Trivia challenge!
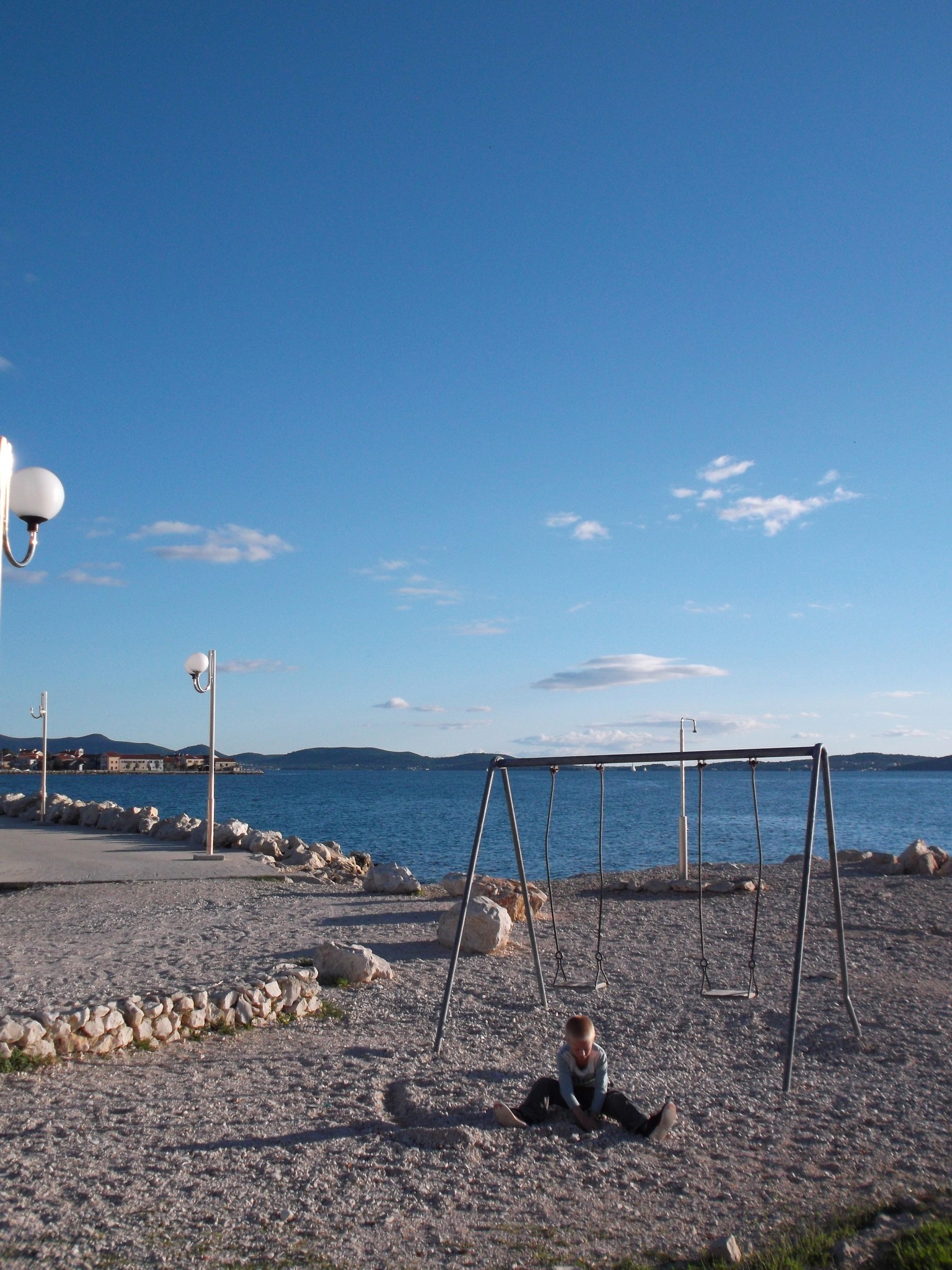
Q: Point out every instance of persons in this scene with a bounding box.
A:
[491,1015,677,1145]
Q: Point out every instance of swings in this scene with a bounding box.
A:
[543,767,610,995]
[698,758,762,1000]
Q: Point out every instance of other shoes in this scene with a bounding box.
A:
[640,1101,677,1143]
[494,1100,529,1129]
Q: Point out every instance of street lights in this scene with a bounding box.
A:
[678,717,698,880]
[184,649,216,855]
